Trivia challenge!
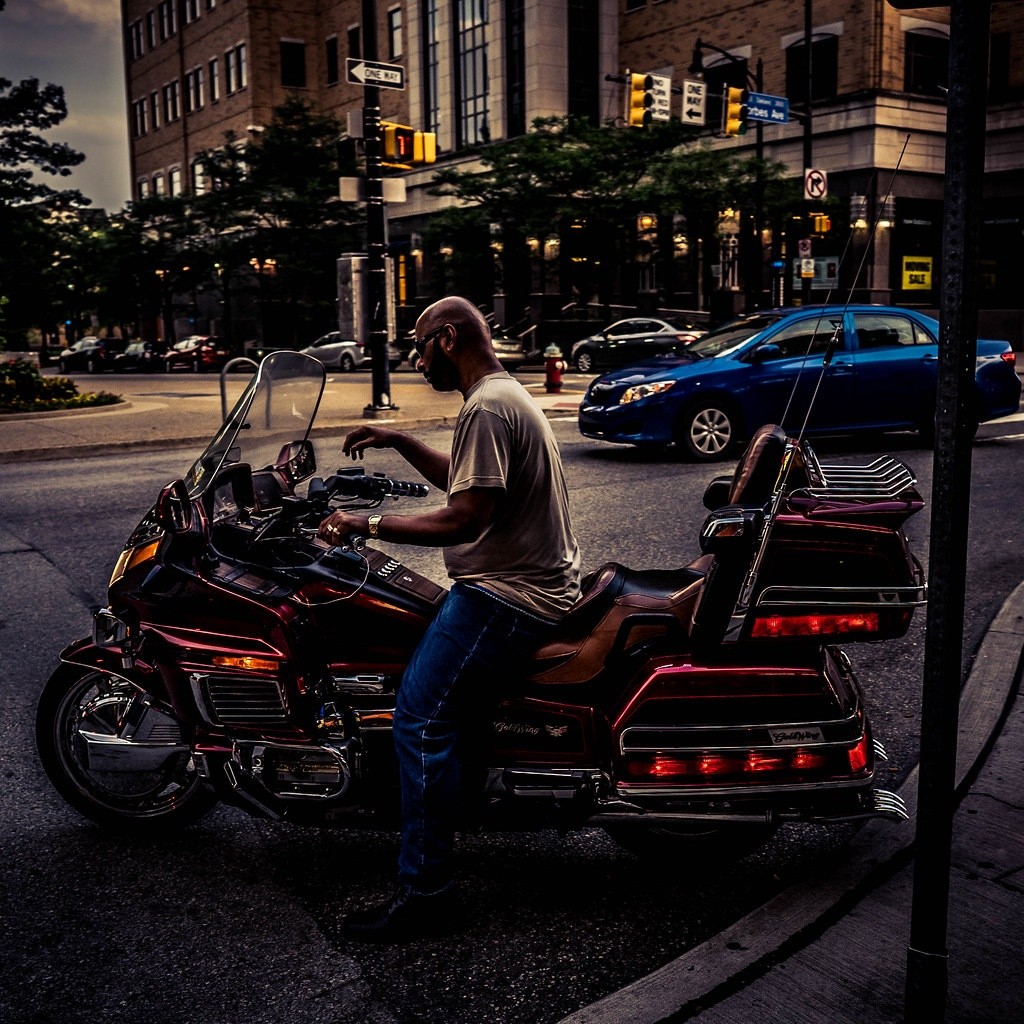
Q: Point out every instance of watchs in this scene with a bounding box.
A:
[367,514,383,539]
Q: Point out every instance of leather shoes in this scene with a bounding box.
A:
[340,883,466,944]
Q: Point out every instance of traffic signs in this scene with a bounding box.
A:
[345,57,407,93]
[683,78,707,125]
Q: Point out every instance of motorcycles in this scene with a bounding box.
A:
[31,346,918,863]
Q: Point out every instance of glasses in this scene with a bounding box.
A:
[412,324,462,357]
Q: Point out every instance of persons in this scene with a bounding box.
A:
[319,295,581,942]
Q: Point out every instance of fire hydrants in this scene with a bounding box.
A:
[541,342,571,393]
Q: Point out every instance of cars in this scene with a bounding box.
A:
[294,332,403,373]
[573,304,1024,458]
[568,315,708,375]
[37,346,67,368]
[162,335,234,374]
[113,340,169,374]
[405,332,527,370]
[58,335,124,374]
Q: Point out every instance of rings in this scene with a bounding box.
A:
[333,527,341,534]
[327,524,334,531]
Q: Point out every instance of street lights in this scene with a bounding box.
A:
[688,36,765,312]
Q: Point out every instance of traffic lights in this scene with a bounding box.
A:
[721,82,751,139]
[815,214,832,233]
[384,126,416,161]
[623,69,658,130]
[413,131,437,163]
